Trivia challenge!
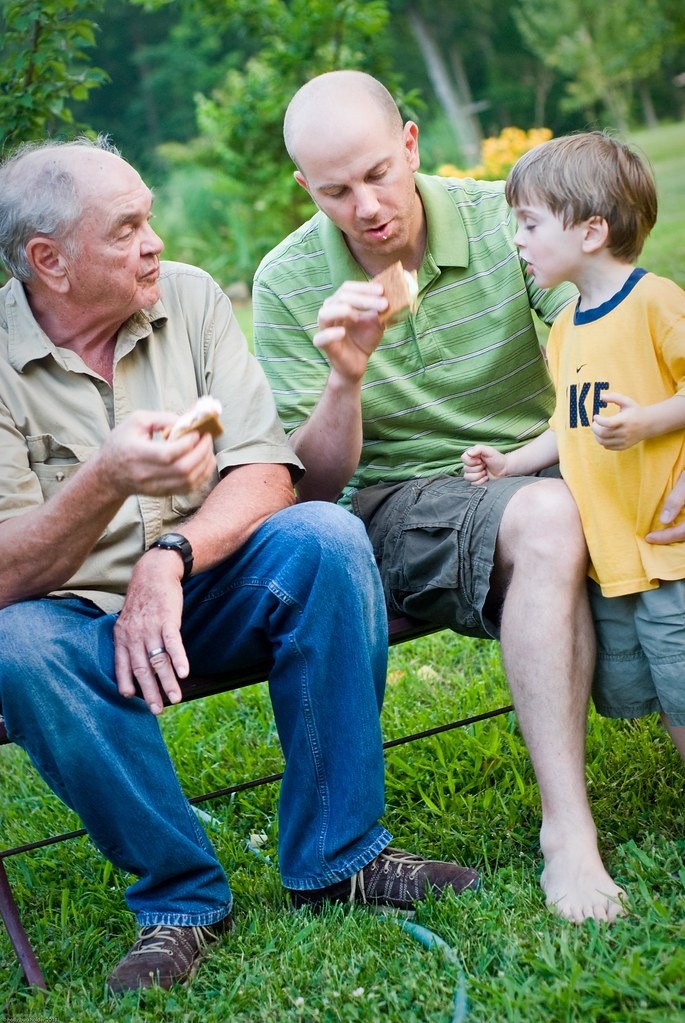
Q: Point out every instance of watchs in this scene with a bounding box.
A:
[148,533,193,583]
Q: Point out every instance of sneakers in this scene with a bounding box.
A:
[108,903,234,998]
[289,845,478,912]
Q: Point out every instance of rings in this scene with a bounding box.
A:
[148,648,167,658]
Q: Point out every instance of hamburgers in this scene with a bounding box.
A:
[372,262,419,324]
[162,393,223,441]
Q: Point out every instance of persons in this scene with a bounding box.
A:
[249,70,685,925]
[0,144,485,990]
[461,126,685,760]
